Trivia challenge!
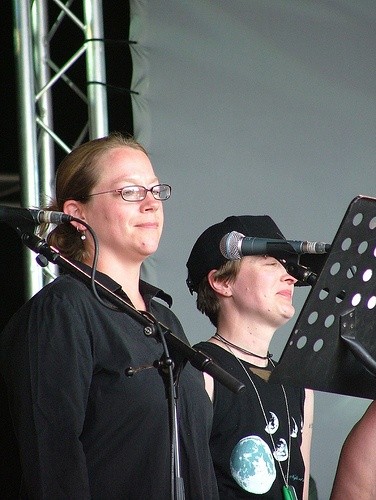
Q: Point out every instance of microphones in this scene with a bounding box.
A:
[220,230,331,261]
[0,205,73,225]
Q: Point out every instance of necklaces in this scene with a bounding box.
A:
[211,329,298,500]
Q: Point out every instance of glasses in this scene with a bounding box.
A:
[79,183,172,203]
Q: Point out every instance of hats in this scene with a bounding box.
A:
[186,215,330,295]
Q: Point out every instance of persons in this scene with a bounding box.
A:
[0,132,222,500]
[328,398,376,500]
[186,214,315,500]
